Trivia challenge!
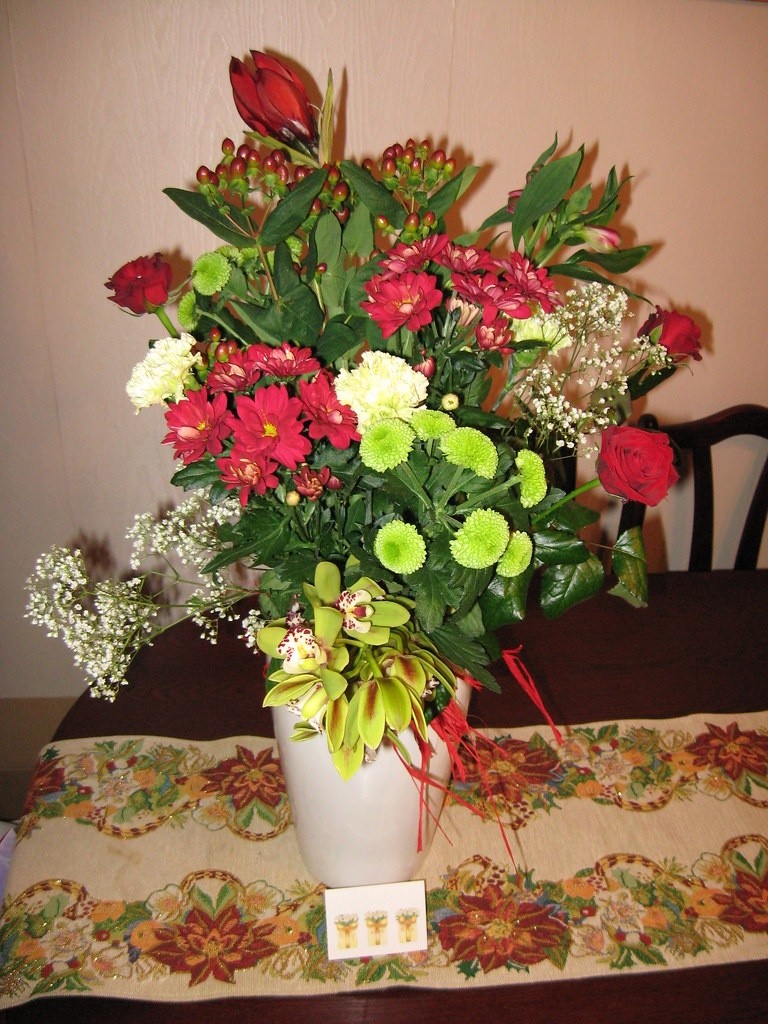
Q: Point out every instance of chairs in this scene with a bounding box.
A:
[616,403,768,569]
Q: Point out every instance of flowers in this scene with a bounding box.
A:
[22,51,707,786]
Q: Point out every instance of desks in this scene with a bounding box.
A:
[0,569,768,1024]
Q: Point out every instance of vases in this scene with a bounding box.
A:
[272,678,469,884]
